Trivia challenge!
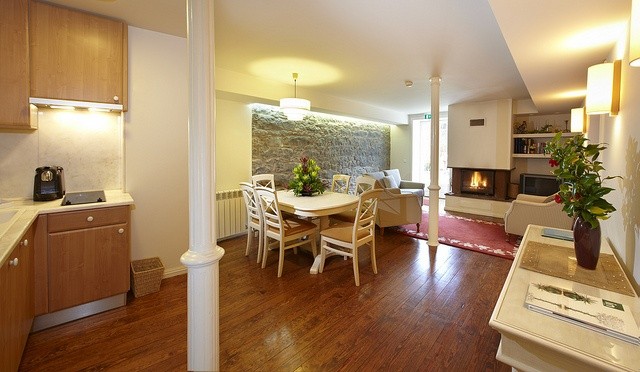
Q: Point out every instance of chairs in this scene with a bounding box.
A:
[310,174,352,251]
[256,189,318,278]
[363,173,421,235]
[316,189,384,286]
[252,174,276,248]
[328,174,375,260]
[238,181,297,263]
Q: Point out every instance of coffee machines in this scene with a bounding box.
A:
[33,165,66,202]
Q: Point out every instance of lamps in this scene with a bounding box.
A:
[585,60,621,117]
[279,73,310,120]
[570,106,586,134]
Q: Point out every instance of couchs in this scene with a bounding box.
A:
[503,188,574,242]
[365,169,424,206]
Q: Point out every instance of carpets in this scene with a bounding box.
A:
[371,212,522,260]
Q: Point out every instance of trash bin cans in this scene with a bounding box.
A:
[130,256,165,298]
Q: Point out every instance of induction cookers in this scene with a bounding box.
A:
[61,190,106,206]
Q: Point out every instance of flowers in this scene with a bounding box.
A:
[543,131,623,229]
[287,155,325,196]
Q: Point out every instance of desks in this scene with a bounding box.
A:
[276,187,360,256]
[487,224,640,372]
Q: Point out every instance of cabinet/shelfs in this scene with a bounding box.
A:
[0,0,39,130]
[27,0,128,113]
[34,190,134,331]
[0,213,39,372]
[513,113,581,198]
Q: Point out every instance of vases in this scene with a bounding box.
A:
[573,213,601,270]
[300,186,313,196]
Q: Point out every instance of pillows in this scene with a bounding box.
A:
[382,175,398,190]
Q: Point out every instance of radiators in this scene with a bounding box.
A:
[216,188,249,243]
